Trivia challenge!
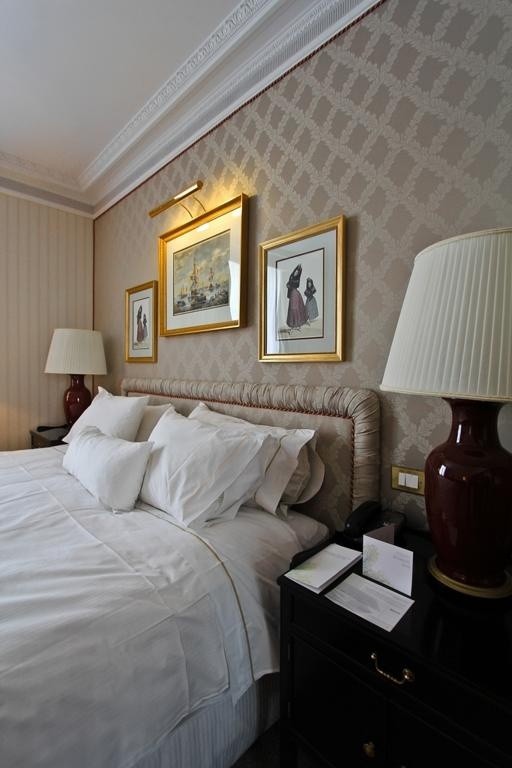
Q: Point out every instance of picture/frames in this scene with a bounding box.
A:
[259,214,346,364]
[124,277,157,364]
[158,192,251,339]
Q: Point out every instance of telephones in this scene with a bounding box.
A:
[344,501,408,548]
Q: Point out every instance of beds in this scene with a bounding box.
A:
[2,376,381,764]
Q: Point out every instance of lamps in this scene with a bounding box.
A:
[373,226,512,600]
[148,180,210,224]
[45,325,110,427]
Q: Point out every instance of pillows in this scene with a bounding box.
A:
[186,401,323,516]
[62,425,155,513]
[60,387,172,442]
[139,406,278,531]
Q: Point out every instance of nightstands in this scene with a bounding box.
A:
[28,422,71,447]
[279,513,511,765]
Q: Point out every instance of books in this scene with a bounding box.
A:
[284,522,415,633]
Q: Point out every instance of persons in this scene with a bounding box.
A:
[286,264,318,332]
[137,306,148,343]
